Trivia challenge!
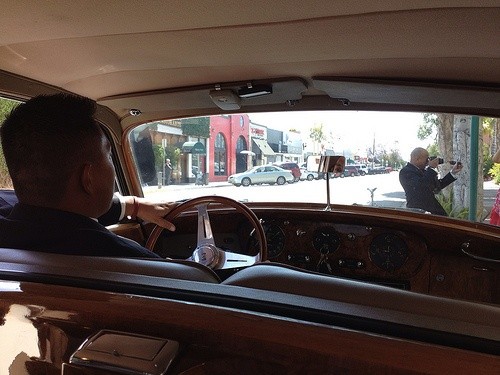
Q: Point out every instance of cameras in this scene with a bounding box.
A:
[429,155,444,167]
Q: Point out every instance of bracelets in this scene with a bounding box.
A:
[127,195,138,220]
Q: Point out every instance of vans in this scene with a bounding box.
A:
[266,162,301,182]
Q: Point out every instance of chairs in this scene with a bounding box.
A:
[0,248,500,328]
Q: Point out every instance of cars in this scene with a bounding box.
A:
[229,165,294,187]
[300,166,319,181]
[318,164,393,181]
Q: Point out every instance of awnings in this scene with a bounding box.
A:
[183,141,206,156]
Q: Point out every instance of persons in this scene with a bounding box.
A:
[489,148,500,226]
[332,156,345,173]
[0,92,182,260]
[164,159,172,186]
[399,147,463,216]
